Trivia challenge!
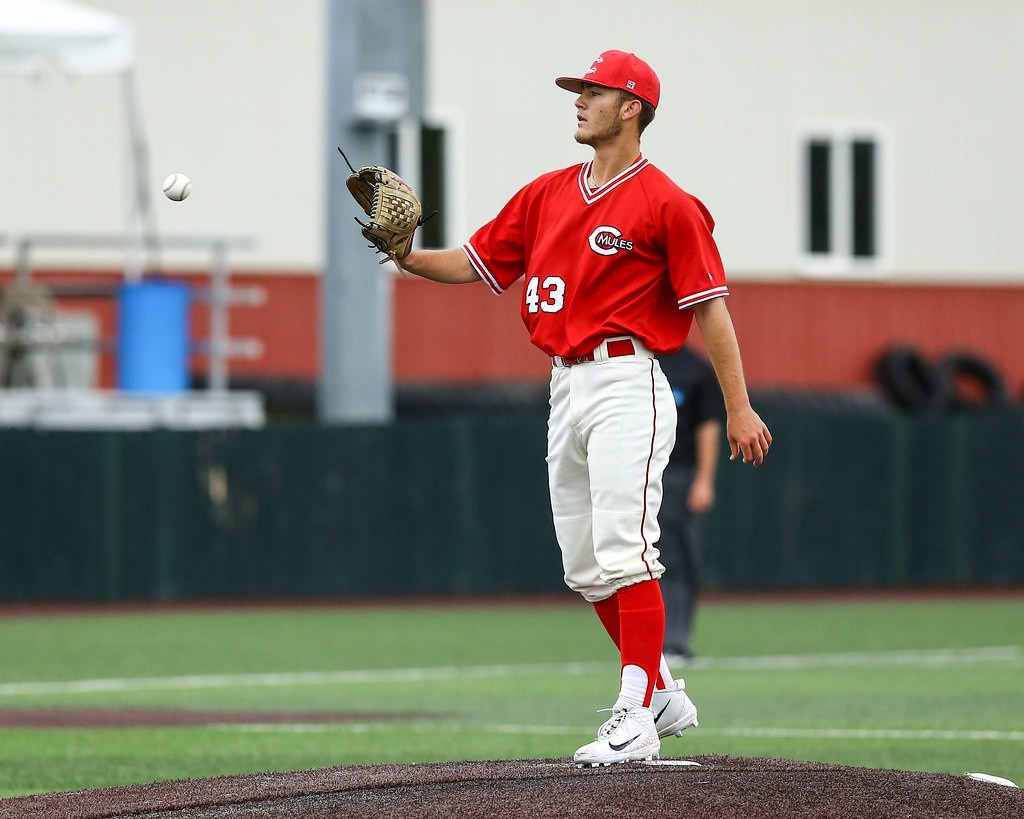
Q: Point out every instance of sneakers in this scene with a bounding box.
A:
[648,678,700,739]
[573,705,661,768]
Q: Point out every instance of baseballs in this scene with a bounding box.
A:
[163,173,192,202]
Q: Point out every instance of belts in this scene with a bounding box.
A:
[550,338,635,367]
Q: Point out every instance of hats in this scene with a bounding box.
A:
[555,49,660,110]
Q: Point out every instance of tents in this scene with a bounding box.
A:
[0,0,160,273]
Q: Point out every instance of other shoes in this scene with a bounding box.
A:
[664,651,691,669]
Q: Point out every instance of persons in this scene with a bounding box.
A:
[364,50,773,764]
[653,339,725,667]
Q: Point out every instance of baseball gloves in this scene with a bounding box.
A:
[346,166,421,260]
